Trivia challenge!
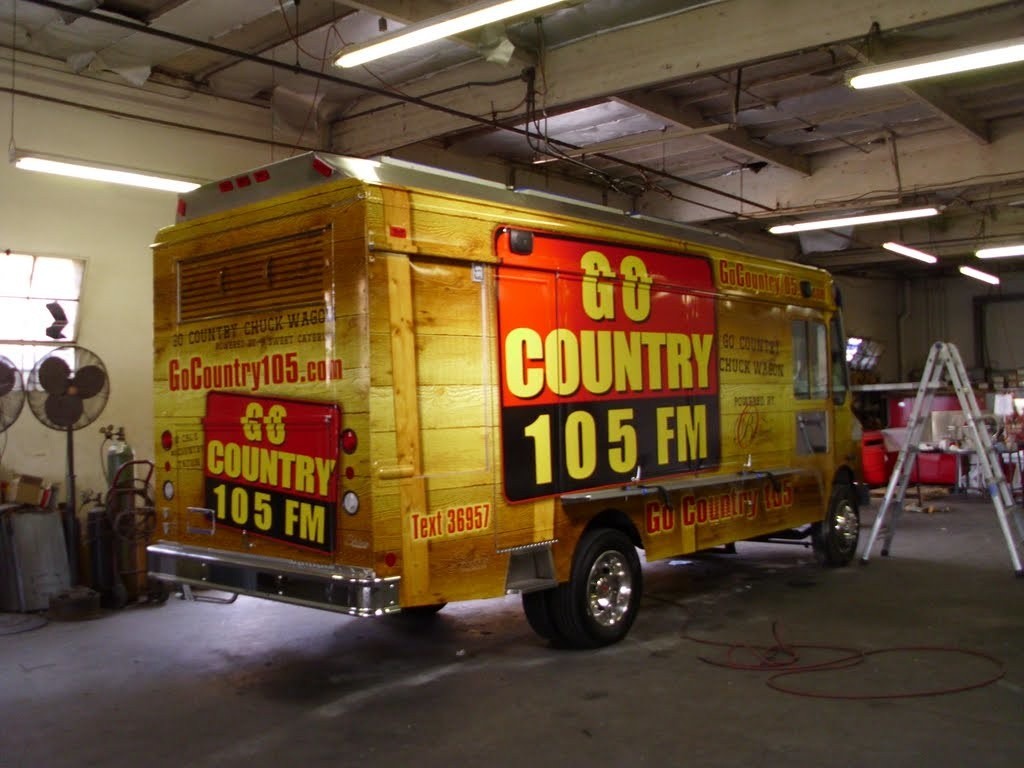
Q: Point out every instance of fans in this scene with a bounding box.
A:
[0,354,46,635]
[26,345,111,586]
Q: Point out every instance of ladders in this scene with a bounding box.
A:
[859,339,1024,580]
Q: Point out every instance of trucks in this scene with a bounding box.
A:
[143,146,883,651]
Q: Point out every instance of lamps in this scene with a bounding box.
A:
[532,66,743,165]
[843,19,1024,90]
[957,255,1000,285]
[4,1,276,193]
[331,0,566,70]
[975,241,1023,259]
[763,202,943,235]
[878,196,941,265]
